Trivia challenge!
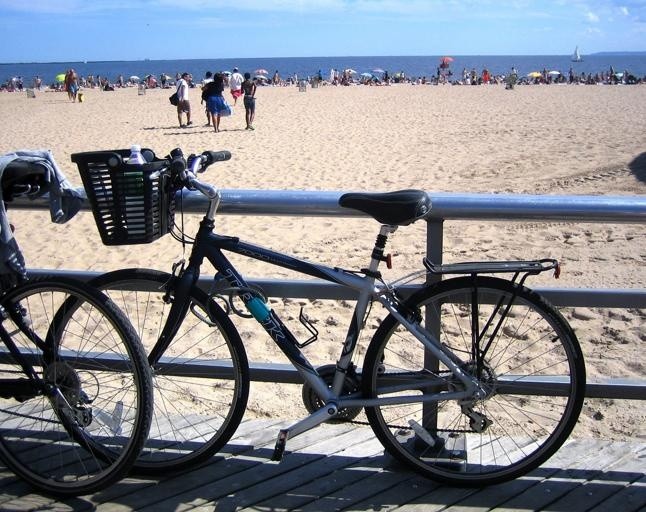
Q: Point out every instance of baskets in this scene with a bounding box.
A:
[71,148,176,245]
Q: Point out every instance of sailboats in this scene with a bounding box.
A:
[570,44,585,63]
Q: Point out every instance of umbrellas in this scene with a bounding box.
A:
[440,57,455,64]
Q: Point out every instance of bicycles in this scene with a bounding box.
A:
[0,159,157,502]
[36,145,590,488]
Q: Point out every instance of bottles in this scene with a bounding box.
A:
[124,146,146,233]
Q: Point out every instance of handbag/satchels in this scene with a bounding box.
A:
[169,92,179,105]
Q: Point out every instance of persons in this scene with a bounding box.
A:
[201,71,214,127]
[240,72,258,131]
[176,73,194,128]
[1,67,645,102]
[201,72,234,134]
